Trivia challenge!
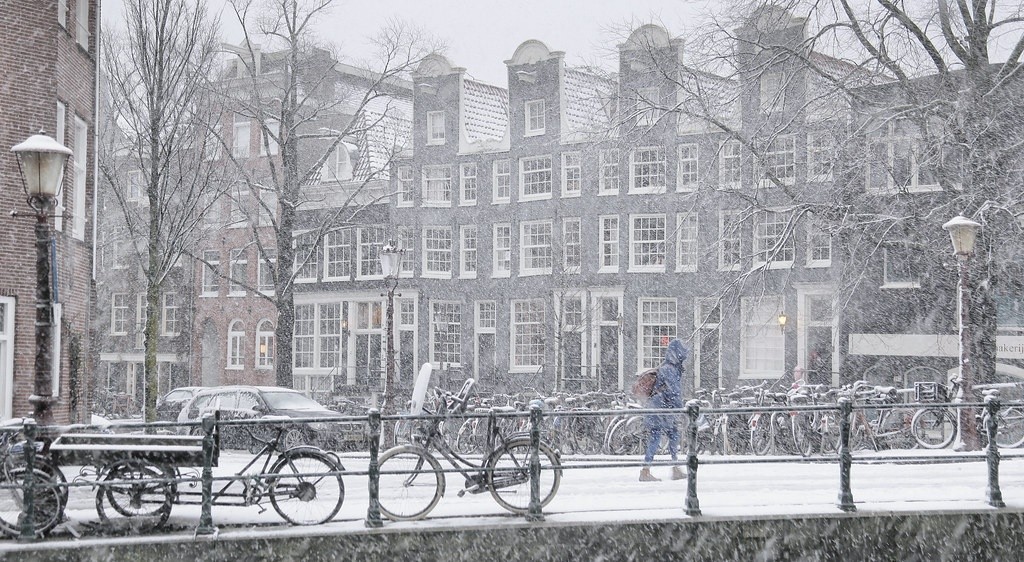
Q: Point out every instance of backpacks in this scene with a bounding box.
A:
[638,368,659,395]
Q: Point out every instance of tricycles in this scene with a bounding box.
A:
[43,407,348,536]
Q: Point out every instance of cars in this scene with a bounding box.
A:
[177,386,363,452]
[157,388,212,424]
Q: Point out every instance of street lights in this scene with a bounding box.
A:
[376,238,406,452]
[9,126,76,456]
[941,212,986,451]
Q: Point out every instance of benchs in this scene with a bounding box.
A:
[50,411,222,485]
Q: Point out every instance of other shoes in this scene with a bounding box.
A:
[673,467,688,479]
[639,470,662,481]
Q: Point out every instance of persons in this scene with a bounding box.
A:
[638,339,688,481]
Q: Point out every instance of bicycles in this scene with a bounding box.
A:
[326,378,924,458]
[910,374,1024,449]
[95,386,146,419]
[0,417,70,541]
[379,380,564,522]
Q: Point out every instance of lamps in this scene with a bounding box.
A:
[341,319,350,337]
[779,312,794,338]
[258,342,266,358]
